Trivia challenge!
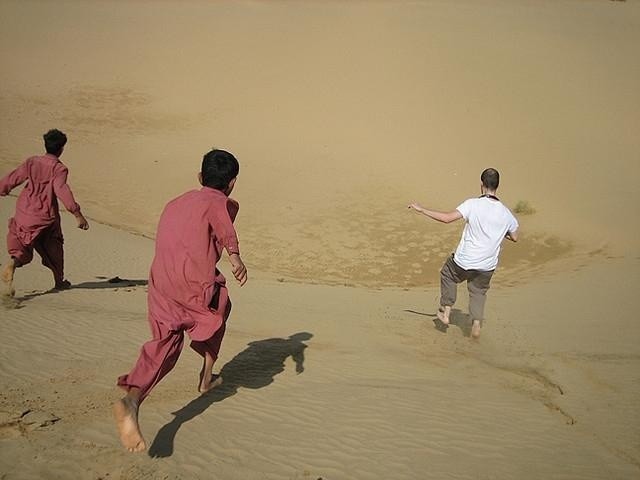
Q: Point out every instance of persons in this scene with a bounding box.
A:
[111,147,248,453]
[0,126,90,291]
[407,168,519,339]
